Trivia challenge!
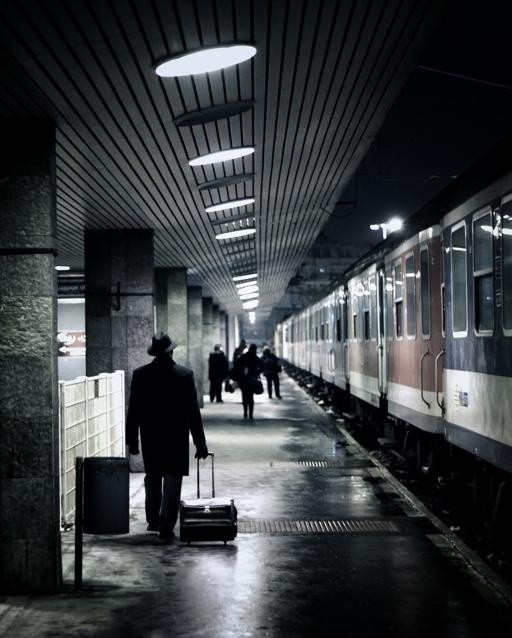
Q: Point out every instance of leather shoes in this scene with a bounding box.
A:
[147,523,175,541]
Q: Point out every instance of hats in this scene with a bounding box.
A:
[147,334,177,356]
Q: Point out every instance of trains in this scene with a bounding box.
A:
[273,146,510,529]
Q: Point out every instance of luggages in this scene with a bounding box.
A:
[179,452,238,544]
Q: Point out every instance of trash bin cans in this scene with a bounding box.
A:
[74,457,129,538]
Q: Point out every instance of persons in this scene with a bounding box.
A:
[259,348,282,399]
[232,339,246,366]
[237,343,265,419]
[125,332,208,542]
[209,343,230,404]
